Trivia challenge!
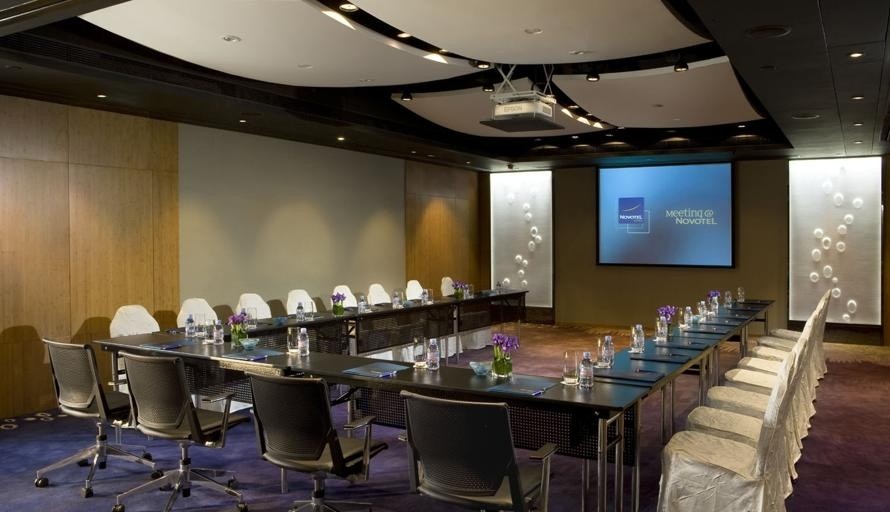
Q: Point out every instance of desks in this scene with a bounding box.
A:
[94,283,776,442]
[152,289,529,368]
[65,333,651,511]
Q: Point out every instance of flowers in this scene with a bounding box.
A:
[226,313,249,328]
[655,304,679,325]
[705,288,721,304]
[331,292,347,303]
[491,332,520,353]
[452,279,469,290]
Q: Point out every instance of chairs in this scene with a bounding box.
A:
[235,292,272,321]
[34,338,164,499]
[244,371,389,512]
[656,351,796,512]
[111,349,249,512]
[440,276,494,350]
[683,338,807,480]
[286,288,317,316]
[366,282,424,363]
[330,285,394,361]
[405,279,464,358]
[399,389,561,512]
[176,297,219,328]
[751,345,791,362]
[704,310,816,440]
[736,288,832,380]
[756,336,798,352]
[769,327,802,342]
[109,304,160,391]
[724,294,826,402]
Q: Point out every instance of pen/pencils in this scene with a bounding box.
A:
[165,344,182,351]
[251,355,268,360]
[690,341,704,344]
[381,371,397,377]
[533,388,546,396]
[667,353,688,357]
[637,369,658,372]
[168,330,182,333]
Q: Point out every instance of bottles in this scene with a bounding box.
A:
[496,281,502,295]
[426,338,440,370]
[710,297,718,318]
[421,289,428,305]
[601,335,615,367]
[464,285,469,300]
[357,296,365,314]
[578,351,594,388]
[240,308,248,331]
[659,316,668,338]
[393,292,399,309]
[296,302,304,321]
[297,328,310,357]
[700,301,708,322]
[186,314,196,337]
[737,288,742,302]
[683,306,693,329]
[634,324,644,354]
[213,320,224,345]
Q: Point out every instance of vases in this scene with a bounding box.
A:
[230,323,248,346]
[332,301,345,315]
[491,355,513,378]
[453,289,465,300]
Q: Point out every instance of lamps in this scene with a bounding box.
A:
[674,62,688,72]
[476,62,488,70]
[477,60,490,69]
[483,79,494,92]
[402,92,414,101]
[587,72,600,82]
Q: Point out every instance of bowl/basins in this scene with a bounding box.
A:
[470,361,493,376]
[238,338,259,350]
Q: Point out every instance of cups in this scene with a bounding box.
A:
[657,323,668,344]
[204,319,216,343]
[413,337,426,366]
[562,351,578,384]
[247,308,257,329]
[597,338,610,366]
[630,327,640,351]
[287,327,301,352]
[194,313,204,336]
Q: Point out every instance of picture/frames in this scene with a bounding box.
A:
[488,170,556,325]
[787,155,885,346]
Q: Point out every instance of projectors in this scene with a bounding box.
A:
[490,91,556,120]
